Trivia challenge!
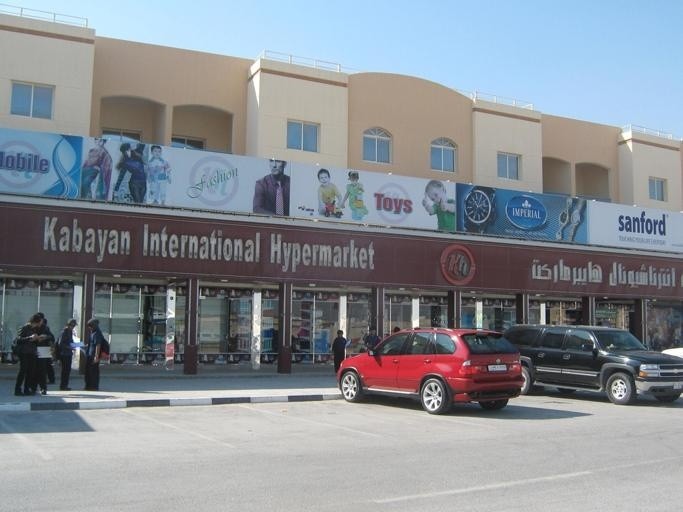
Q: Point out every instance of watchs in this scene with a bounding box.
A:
[463,186,498,233]
[555,197,573,241]
[567,199,585,242]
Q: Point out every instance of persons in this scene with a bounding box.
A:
[81,138,172,205]
[339,171,368,220]
[422,180,456,231]
[332,330,347,376]
[84,319,104,391]
[318,169,344,215]
[15,313,55,395]
[365,327,382,352]
[58,319,78,390]
[253,160,290,216]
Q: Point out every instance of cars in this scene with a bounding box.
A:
[661,347,683,358]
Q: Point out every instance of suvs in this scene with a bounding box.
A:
[503,319,682,407]
[335,326,526,414]
